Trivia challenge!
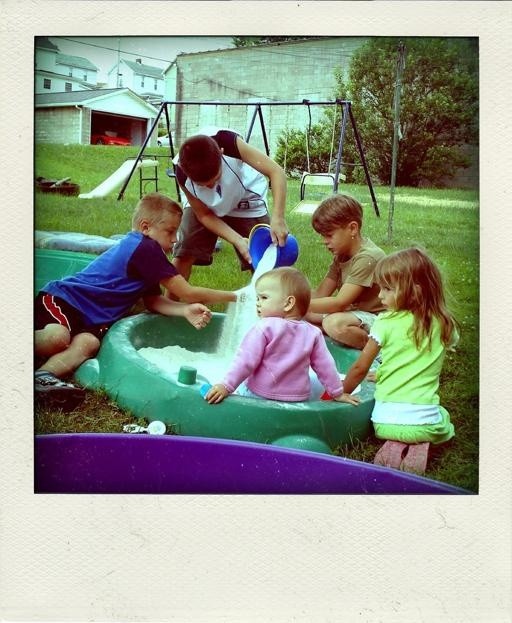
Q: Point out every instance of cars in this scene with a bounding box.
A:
[156,130,179,147]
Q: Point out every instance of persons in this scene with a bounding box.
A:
[341,247,462,476]
[33,194,247,408]
[206,267,361,406]
[162,129,290,305]
[303,193,388,350]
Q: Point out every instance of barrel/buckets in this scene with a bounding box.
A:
[247,223,298,275]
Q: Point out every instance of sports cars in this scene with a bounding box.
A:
[92,128,133,149]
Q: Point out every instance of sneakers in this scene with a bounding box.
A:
[373,440,407,470]
[34,370,85,412]
[399,443,429,476]
